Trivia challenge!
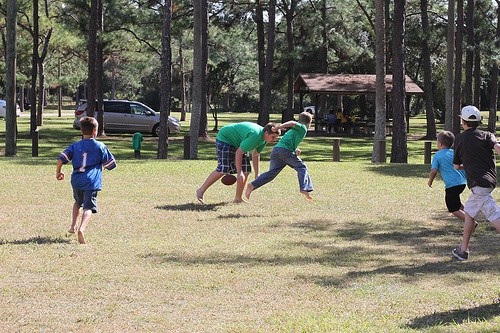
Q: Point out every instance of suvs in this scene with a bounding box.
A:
[72,100,182,137]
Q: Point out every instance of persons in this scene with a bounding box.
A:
[427,131,479,231]
[327,109,356,133]
[245,111,314,200]
[452,106,500,261]
[56,116,117,244]
[196,121,281,204]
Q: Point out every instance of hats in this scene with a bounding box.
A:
[458,106,481,121]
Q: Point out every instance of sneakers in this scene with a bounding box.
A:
[452,248,469,261]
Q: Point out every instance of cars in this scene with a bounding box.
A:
[0,99,22,119]
[303,106,320,113]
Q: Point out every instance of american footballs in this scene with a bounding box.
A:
[221,175,237,186]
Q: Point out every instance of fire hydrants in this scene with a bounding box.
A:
[132,132,143,160]
